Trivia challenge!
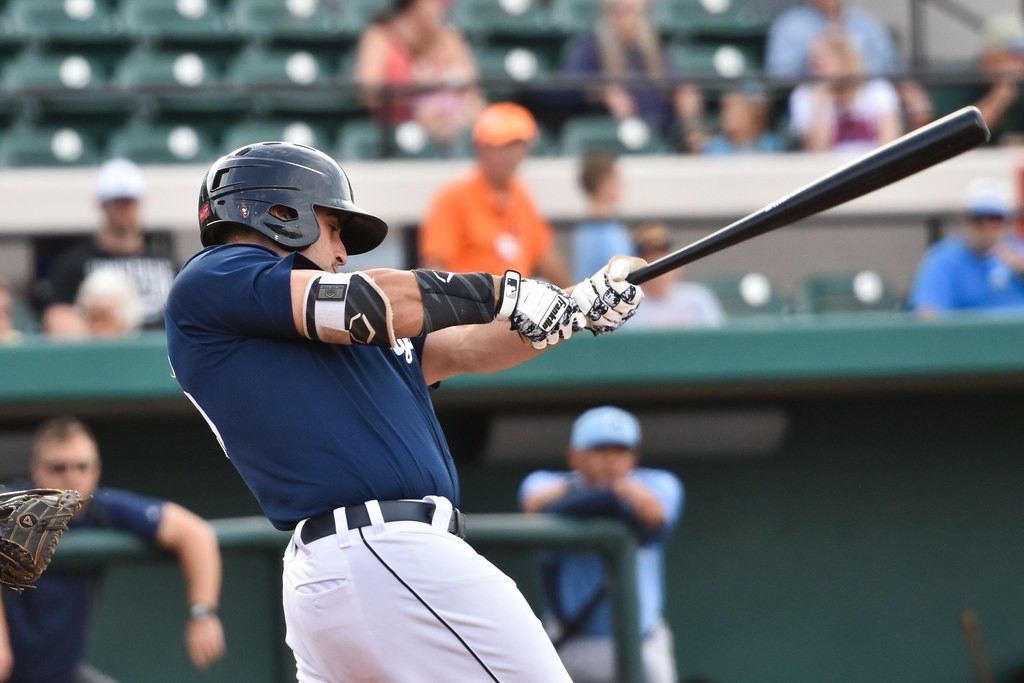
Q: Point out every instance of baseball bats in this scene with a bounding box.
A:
[627,106,991,285]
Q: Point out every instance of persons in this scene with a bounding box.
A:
[0,413,228,683]
[408,101,572,297]
[517,406,686,683]
[163,140,649,683]
[566,149,638,293]
[26,156,183,341]
[352,2,1023,159]
[608,219,729,329]
[902,182,1024,316]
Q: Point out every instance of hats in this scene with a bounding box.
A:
[476,102,537,146]
[98,158,143,202]
[571,406,641,448]
[966,181,1013,216]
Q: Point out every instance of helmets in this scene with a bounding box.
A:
[198,140,389,255]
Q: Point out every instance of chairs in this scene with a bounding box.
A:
[558,117,673,155]
[707,275,796,316]
[104,125,216,166]
[673,45,759,75]
[221,118,330,158]
[808,273,902,314]
[0,126,101,168]
[0,36,110,93]
[111,38,221,88]
[0,0,769,53]
[222,41,333,84]
[471,46,555,80]
[335,116,441,159]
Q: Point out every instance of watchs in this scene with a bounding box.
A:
[186,603,219,623]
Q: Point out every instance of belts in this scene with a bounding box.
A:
[294,501,468,556]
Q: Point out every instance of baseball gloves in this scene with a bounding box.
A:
[0,488,85,590]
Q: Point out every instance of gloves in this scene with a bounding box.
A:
[572,256,649,336]
[494,270,586,349]
